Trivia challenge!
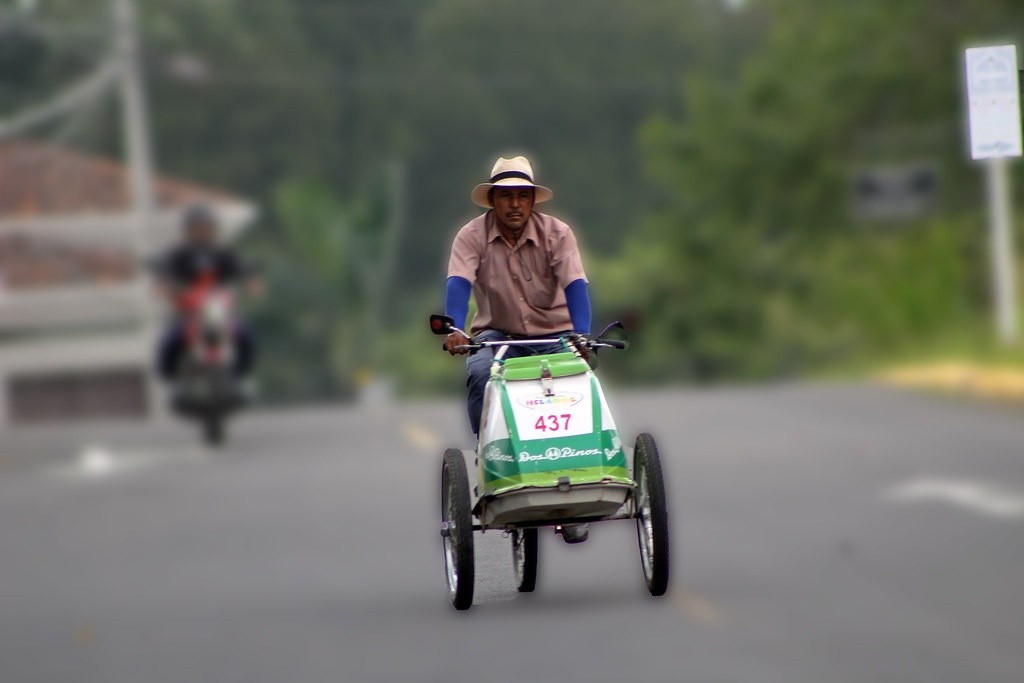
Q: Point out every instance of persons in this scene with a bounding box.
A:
[446,155,595,545]
[145,203,256,383]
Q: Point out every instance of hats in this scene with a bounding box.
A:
[472,156,553,209]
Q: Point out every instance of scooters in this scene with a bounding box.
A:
[157,251,257,444]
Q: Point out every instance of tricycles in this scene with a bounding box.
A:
[428,312,669,610]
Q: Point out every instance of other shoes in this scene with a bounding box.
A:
[562,523,588,542]
